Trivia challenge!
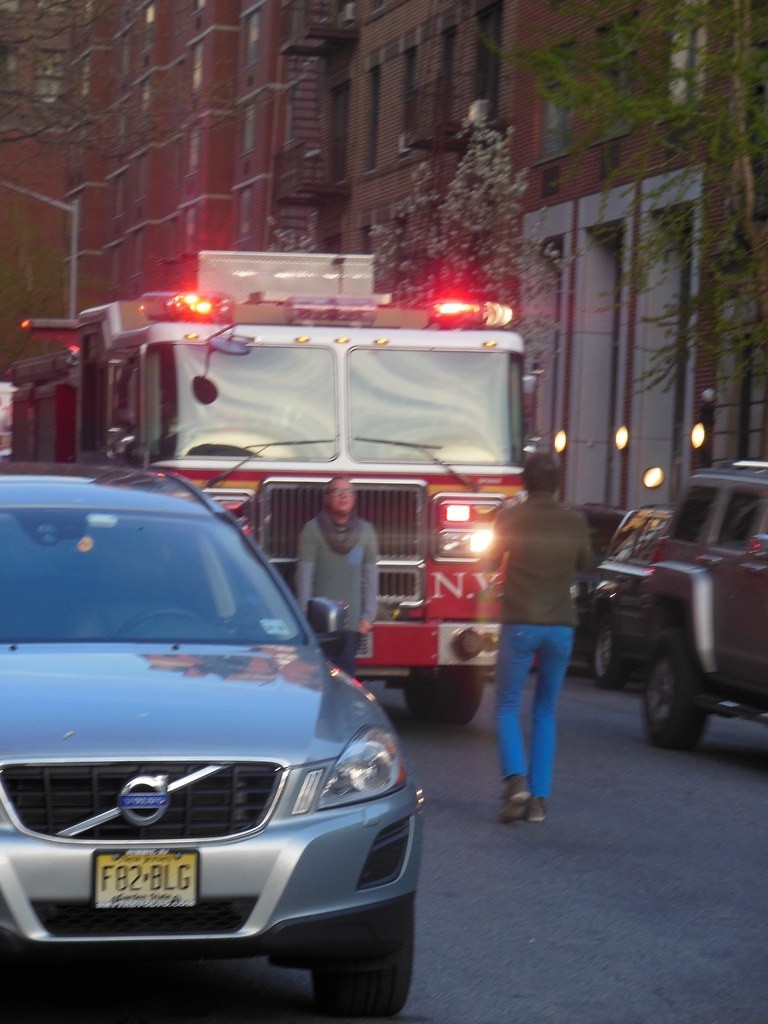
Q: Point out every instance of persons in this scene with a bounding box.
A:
[476,453,594,823]
[294,476,378,677]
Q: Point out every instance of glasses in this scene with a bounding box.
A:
[327,486,354,497]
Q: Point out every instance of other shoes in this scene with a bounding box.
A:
[499,776,531,822]
[526,797,549,822]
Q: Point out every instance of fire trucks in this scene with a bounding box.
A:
[7,249,527,729]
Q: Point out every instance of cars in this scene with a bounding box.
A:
[638,458,768,751]
[572,502,678,690]
[0,461,425,1017]
